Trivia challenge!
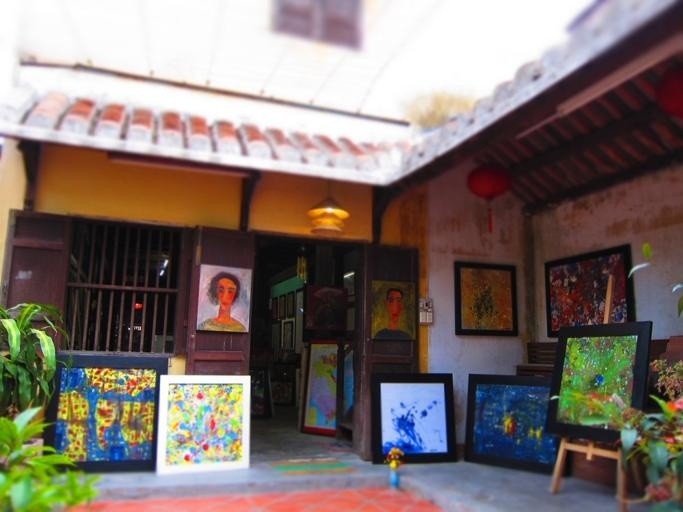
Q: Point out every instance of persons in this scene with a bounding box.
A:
[375,288,413,338]
[198,272,247,331]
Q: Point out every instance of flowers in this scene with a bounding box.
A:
[384,448,405,469]
[549,359,683,502]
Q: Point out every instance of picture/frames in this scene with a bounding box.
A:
[271,287,304,355]
[301,340,354,436]
[156,375,251,475]
[370,373,457,464]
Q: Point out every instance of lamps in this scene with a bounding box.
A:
[307,179,350,234]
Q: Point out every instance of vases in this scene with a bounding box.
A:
[390,470,399,487]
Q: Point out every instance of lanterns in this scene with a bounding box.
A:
[465,164,514,231]
